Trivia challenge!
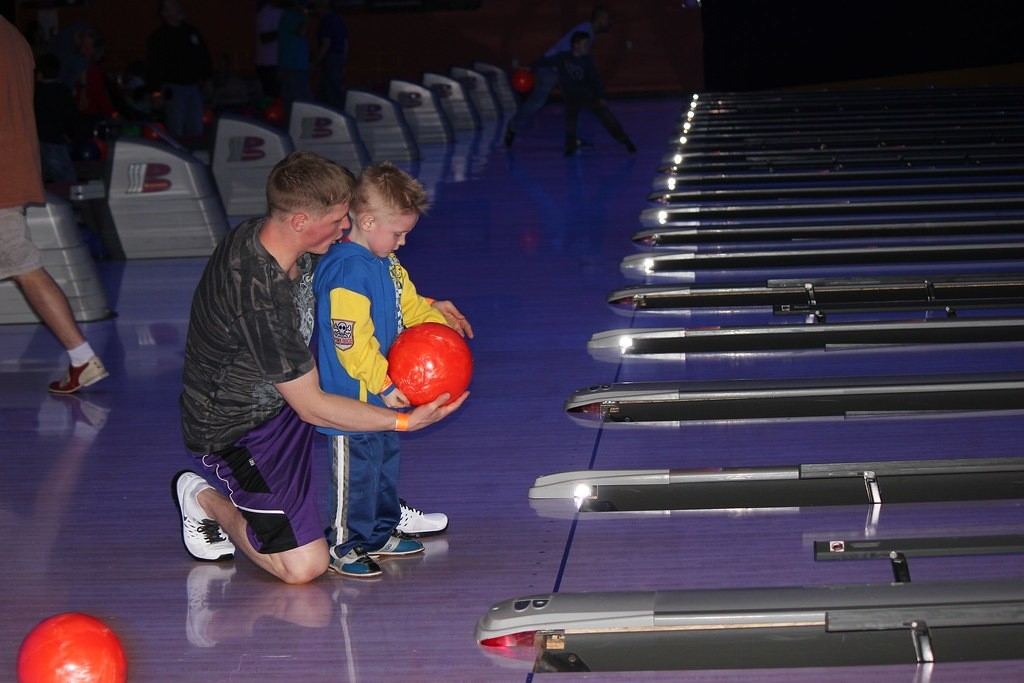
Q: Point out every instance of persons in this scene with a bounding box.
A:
[1,14,109,393]
[170,150,474,584]
[277,0,311,124]
[309,162,454,577]
[311,0,348,113]
[504,7,613,147]
[145,0,212,136]
[258,0,287,131]
[518,30,637,158]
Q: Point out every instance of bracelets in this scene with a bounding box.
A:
[394,411,408,432]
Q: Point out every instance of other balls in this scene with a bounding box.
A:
[90,138,108,161]
[267,105,286,122]
[201,108,214,125]
[16,613,129,683]
[512,69,535,92]
[387,322,473,407]
[144,121,167,141]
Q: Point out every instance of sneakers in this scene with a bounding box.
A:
[47,353,109,393]
[328,546,382,577]
[170,467,235,561]
[369,535,426,559]
[393,502,449,541]
